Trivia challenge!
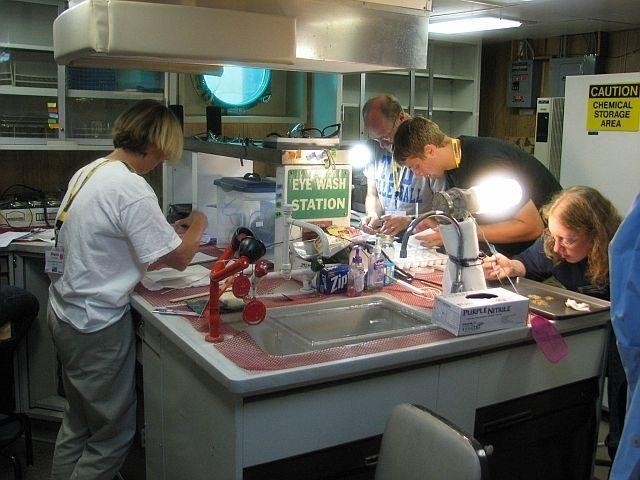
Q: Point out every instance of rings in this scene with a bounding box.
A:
[391,226,395,231]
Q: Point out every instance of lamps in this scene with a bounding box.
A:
[432,167,525,293]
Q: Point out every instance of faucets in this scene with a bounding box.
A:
[399,210,465,293]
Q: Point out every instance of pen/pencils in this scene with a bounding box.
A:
[489,244,497,269]
[152,307,204,317]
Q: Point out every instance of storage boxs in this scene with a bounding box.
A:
[213,176,274,259]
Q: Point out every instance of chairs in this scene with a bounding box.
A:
[0,285,41,479]
[375,402,495,480]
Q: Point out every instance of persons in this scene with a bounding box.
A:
[393,117,565,260]
[483,185,626,462]
[42,98,208,480]
[359,94,445,237]
[609,193,639,480]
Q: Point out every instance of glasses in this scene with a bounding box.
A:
[367,114,398,143]
[544,231,590,247]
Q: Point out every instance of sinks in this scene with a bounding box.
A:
[221,295,438,357]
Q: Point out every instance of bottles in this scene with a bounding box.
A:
[382,234,395,286]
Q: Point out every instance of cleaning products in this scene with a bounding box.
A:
[380,233,396,286]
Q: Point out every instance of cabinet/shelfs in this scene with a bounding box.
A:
[14,251,69,421]
[116,306,163,480]
[0,43,172,150]
[0,248,15,288]
[309,33,483,144]
